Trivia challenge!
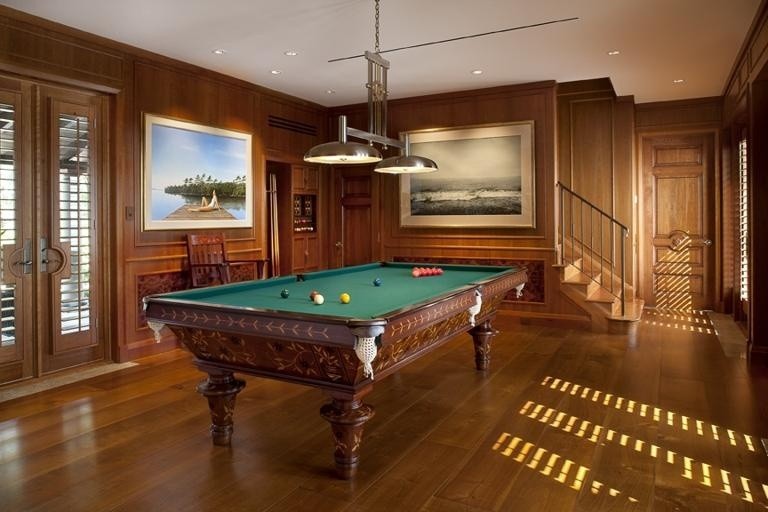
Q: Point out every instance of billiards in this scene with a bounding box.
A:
[412,267,443,277]
[310,291,324,305]
[340,293,351,303]
[281,289,289,297]
[374,278,383,286]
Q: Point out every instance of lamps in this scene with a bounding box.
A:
[303,0,439,175]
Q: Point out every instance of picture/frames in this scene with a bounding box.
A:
[139,111,254,232]
[397,119,536,230]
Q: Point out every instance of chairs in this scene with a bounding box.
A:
[188,232,268,289]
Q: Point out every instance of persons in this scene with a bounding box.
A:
[187,189,221,212]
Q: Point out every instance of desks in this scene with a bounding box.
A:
[142,260,529,479]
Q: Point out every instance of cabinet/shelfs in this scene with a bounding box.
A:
[280,165,322,274]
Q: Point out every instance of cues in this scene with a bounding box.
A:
[270,174,280,277]
